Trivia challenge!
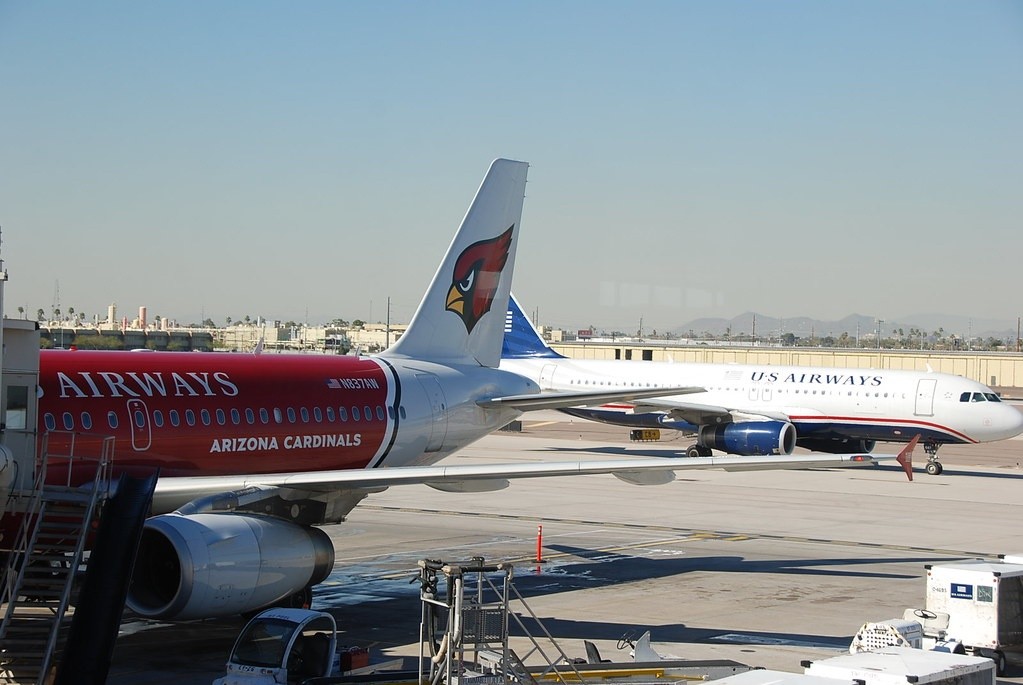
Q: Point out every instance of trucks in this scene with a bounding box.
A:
[851,558,1023,675]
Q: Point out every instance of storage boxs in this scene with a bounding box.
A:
[340,647,369,672]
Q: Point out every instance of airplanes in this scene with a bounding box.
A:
[501,291,1023,476]
[0,157,922,685]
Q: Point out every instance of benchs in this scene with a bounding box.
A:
[298,632,330,677]
